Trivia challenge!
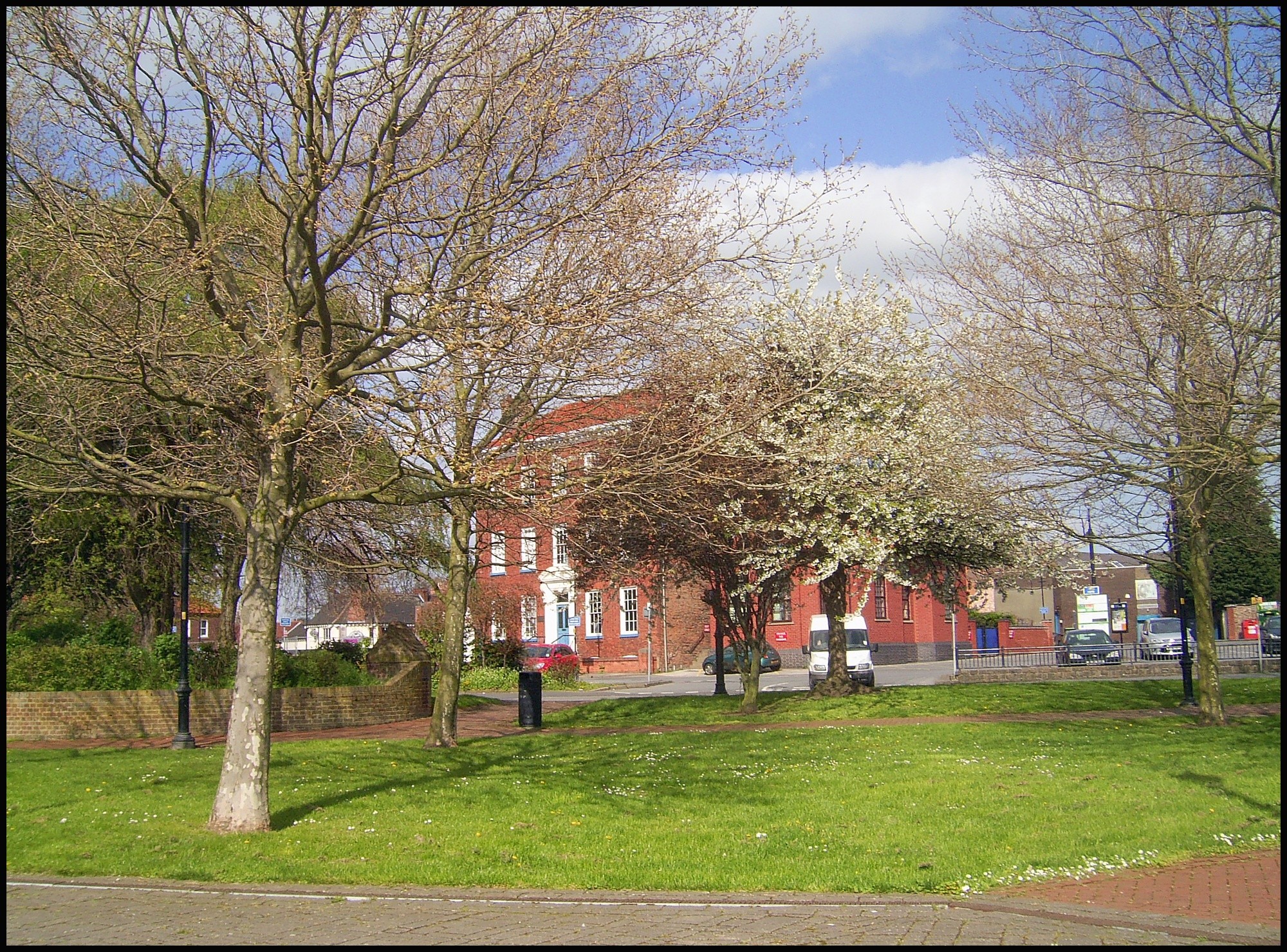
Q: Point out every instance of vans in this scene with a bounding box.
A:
[1139,617,1195,660]
[802,612,878,692]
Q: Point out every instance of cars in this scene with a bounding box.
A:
[1055,629,1121,666]
[702,639,781,675]
[1266,615,1280,653]
[515,643,579,673]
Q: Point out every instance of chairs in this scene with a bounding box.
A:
[1070,638,1078,642]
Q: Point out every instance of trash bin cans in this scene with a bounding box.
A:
[1241,619,1259,639]
[518,672,543,728]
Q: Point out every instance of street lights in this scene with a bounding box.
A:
[1156,427,1198,707]
[1083,494,1097,585]
